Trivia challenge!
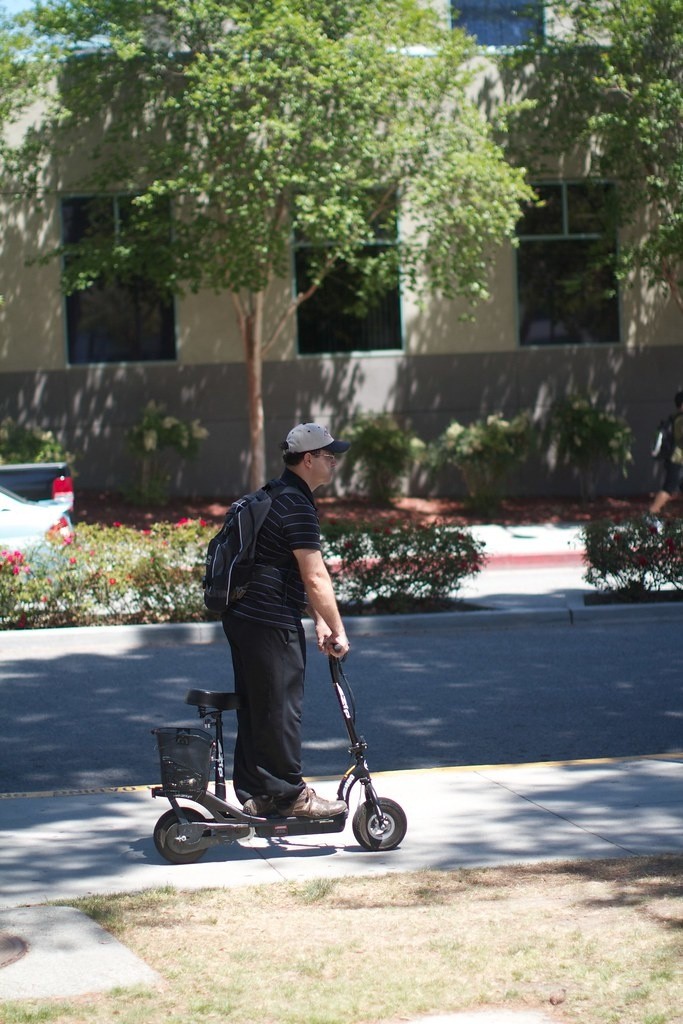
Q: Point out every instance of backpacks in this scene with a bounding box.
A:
[203,484,312,614]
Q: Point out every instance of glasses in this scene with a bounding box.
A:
[309,452,336,463]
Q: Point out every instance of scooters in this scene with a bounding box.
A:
[152,643,408,865]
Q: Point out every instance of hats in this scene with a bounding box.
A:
[284,423,351,458]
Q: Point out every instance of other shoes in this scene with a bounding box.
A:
[244,796,278,816]
[278,786,347,819]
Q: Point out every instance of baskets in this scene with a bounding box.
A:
[151,726,212,795]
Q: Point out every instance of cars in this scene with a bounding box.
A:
[0,463,78,579]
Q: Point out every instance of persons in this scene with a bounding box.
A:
[648,391,683,518]
[222,423,351,821]
